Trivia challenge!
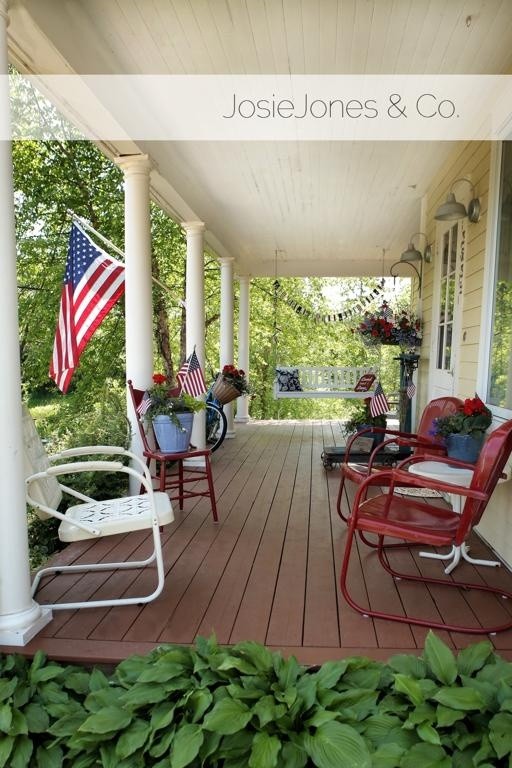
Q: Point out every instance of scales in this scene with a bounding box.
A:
[321,349,420,472]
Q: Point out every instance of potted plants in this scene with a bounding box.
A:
[341,396,387,450]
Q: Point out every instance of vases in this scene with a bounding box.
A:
[147,413,194,453]
[445,433,484,465]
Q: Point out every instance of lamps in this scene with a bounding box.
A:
[401,232,431,262]
[434,179,479,222]
[22,403,174,609]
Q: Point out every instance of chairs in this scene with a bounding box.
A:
[128,380,219,532]
[335,396,464,549]
[340,419,512,636]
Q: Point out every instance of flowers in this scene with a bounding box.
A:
[223,365,250,396]
[432,392,494,438]
[144,374,207,435]
[351,300,423,347]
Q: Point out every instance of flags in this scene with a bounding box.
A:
[136,392,151,414]
[46,222,127,397]
[368,383,391,418]
[406,378,416,399]
[176,352,208,397]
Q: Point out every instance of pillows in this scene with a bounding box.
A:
[275,367,303,393]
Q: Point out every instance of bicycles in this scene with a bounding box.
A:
[205,363,227,453]
